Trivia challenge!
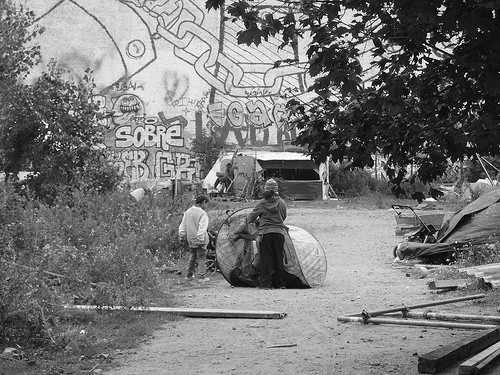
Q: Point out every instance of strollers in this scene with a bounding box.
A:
[392,203,443,260]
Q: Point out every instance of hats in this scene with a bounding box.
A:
[264,178,279,192]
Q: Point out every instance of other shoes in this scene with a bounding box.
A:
[184,275,195,280]
[199,277,210,282]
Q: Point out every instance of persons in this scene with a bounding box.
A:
[169,170,177,199]
[174,195,212,283]
[213,172,227,197]
[247,177,291,288]
[224,163,239,199]
